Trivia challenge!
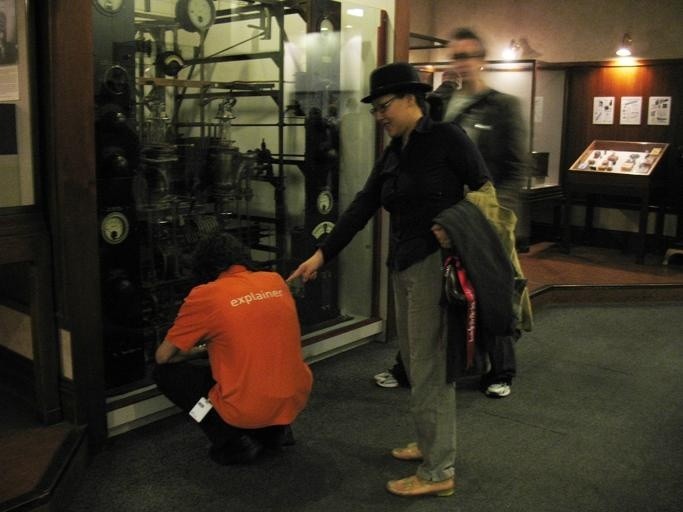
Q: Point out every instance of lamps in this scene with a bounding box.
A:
[616,33,632,57]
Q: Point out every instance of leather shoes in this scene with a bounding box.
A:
[391,443,424,459]
[387,474,455,497]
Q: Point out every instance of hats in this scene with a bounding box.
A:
[360,62,433,102]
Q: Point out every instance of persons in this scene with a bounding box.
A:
[285,62,498,498]
[261,142,272,171]
[152,233,314,466]
[372,29,528,397]
[303,103,338,211]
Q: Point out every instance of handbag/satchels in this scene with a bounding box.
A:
[444,264,467,306]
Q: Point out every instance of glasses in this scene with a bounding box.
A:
[370,94,397,112]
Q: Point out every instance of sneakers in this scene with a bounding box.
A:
[486,383,511,397]
[374,372,400,387]
[208,436,257,464]
[261,425,294,445]
